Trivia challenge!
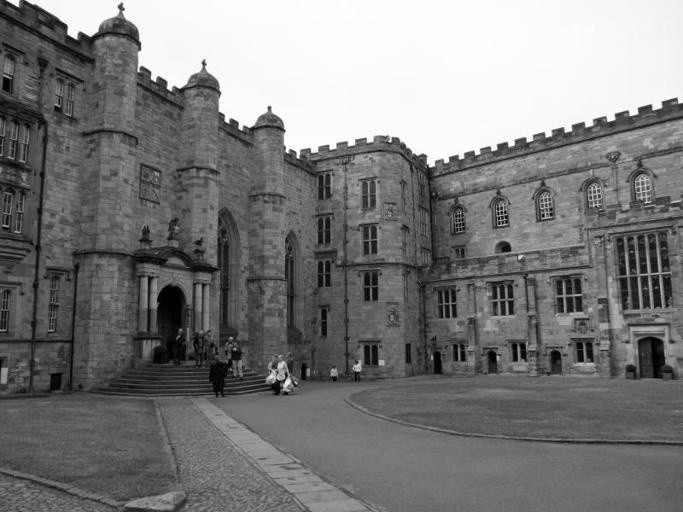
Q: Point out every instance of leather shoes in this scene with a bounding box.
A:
[215,394,225,397]
[234,375,244,380]
[273,392,288,395]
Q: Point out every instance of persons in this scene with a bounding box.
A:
[173,328,243,380]
[330,365,338,382]
[352,360,361,382]
[209,356,228,397]
[268,352,294,395]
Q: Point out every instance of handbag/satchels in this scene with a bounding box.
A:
[265,371,301,392]
